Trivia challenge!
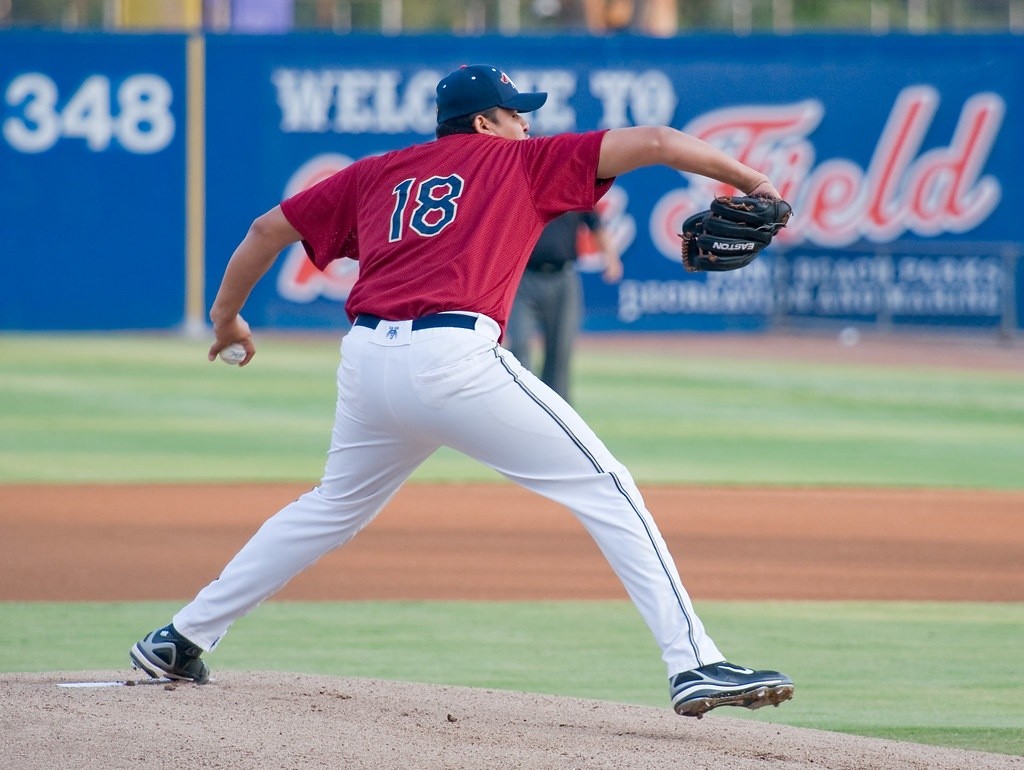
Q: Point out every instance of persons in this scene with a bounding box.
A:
[508,211,622,403]
[129,65,794,718]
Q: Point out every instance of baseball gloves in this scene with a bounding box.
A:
[675,190,796,276]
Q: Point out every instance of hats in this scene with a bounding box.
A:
[436,64,547,124]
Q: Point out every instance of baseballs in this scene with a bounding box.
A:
[217,341,249,366]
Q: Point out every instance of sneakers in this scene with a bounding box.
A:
[669,661,794,719]
[130,623,210,685]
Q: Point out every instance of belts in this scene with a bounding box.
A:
[354,313,478,332]
